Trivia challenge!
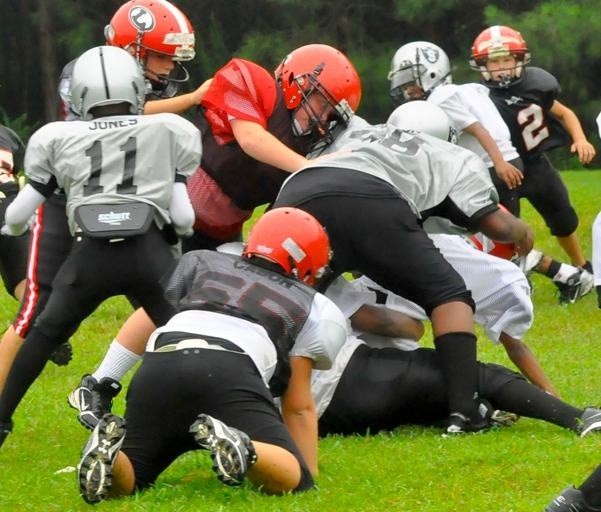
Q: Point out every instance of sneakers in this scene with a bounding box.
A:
[547,483,596,512]
[50,342,72,366]
[188,414,247,487]
[77,413,127,505]
[554,263,594,306]
[65,375,120,429]
[445,401,491,435]
[578,408,601,439]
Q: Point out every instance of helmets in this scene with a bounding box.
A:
[103,0,197,97]
[386,41,453,94]
[468,26,532,87]
[70,46,146,117]
[274,44,363,127]
[241,207,332,285]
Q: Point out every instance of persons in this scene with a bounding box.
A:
[1,1,601,512]
